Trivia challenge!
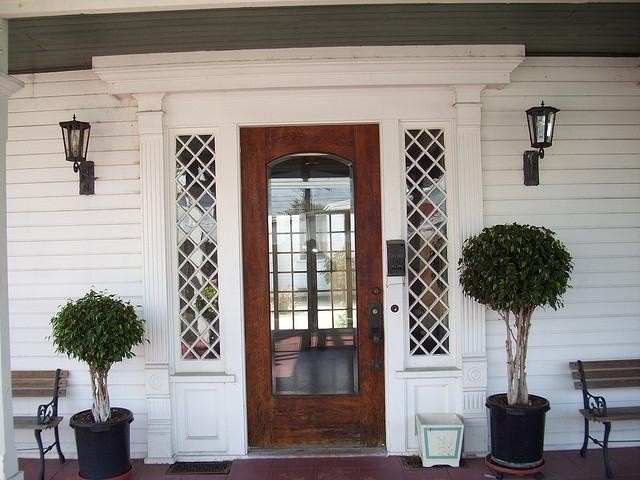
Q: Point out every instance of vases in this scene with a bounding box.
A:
[415,412,466,469]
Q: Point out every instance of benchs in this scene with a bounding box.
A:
[9,368,72,480]
[569,358,640,476]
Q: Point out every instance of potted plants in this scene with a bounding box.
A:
[457,220,573,475]
[47,290,152,476]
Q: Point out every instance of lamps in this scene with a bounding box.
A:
[521,100,560,187]
[59,113,98,198]
